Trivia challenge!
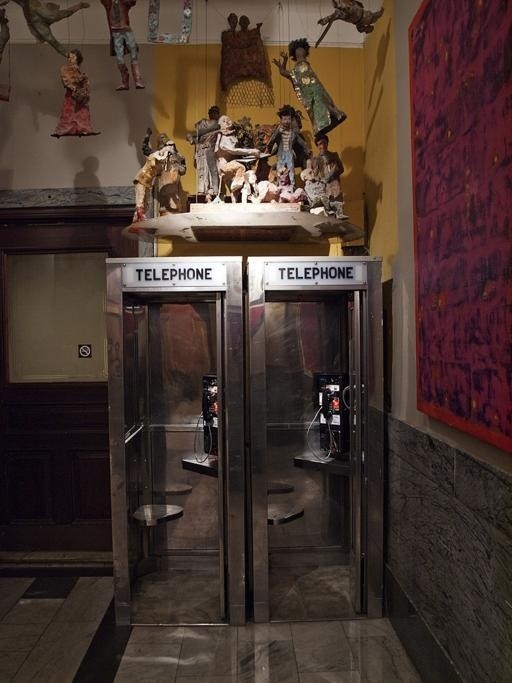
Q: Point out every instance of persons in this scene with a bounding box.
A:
[272,38,347,137]
[317,2,384,34]
[239,15,252,32]
[50,49,101,139]
[16,2,90,58]
[223,13,238,32]
[186,105,351,221]
[2,2,10,102]
[101,2,146,90]
[132,128,187,222]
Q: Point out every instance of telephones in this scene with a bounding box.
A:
[193,374,218,463]
[305,371,350,461]
